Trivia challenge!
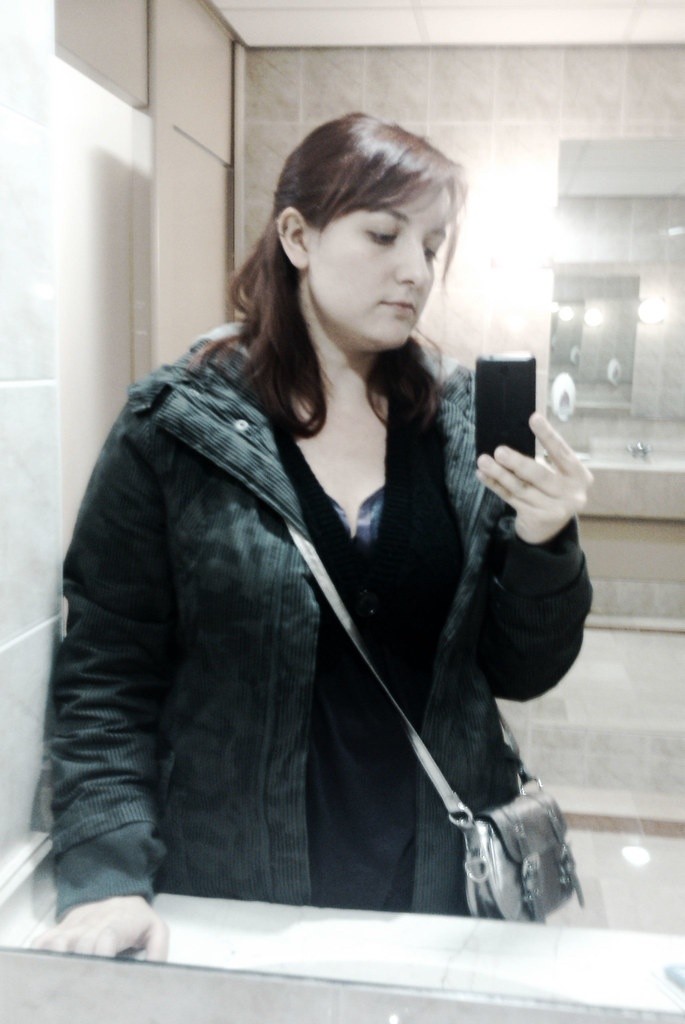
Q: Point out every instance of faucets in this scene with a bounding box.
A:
[626,442,651,457]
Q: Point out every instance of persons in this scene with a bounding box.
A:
[38,113,594,965]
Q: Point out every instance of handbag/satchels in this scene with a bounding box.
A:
[463,779,586,924]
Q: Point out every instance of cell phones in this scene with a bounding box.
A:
[475,354,536,464]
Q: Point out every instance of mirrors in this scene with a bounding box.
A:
[244,44,684,445]
[0,0,684,1018]
